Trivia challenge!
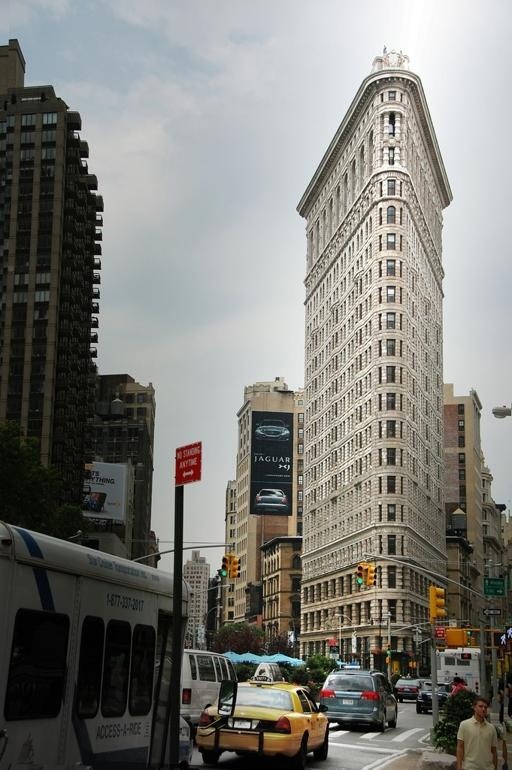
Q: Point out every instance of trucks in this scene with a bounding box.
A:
[432,648,495,708]
[0,516,199,770]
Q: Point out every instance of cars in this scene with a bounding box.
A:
[394,673,455,714]
[254,417,291,443]
[194,660,333,770]
[254,486,290,513]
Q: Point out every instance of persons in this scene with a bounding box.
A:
[495,686,506,723]
[505,680,512,720]
[450,677,467,695]
[455,698,499,770]
[461,678,472,692]
[391,670,402,703]
[399,673,420,680]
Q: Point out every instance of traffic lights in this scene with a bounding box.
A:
[428,585,448,626]
[386,657,390,664]
[491,406,512,419]
[356,563,365,587]
[221,555,230,579]
[366,564,377,587]
[466,626,472,646]
[229,555,241,580]
[499,633,507,648]
[387,644,391,655]
[504,625,512,639]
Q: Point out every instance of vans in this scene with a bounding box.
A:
[178,648,241,736]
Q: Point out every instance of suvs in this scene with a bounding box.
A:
[315,664,404,735]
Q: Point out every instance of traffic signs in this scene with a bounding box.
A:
[435,628,445,640]
[174,439,204,486]
[483,577,507,597]
[483,608,503,616]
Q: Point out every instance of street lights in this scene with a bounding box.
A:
[194,583,234,598]
[334,613,356,668]
[413,626,424,675]
[204,605,225,625]
